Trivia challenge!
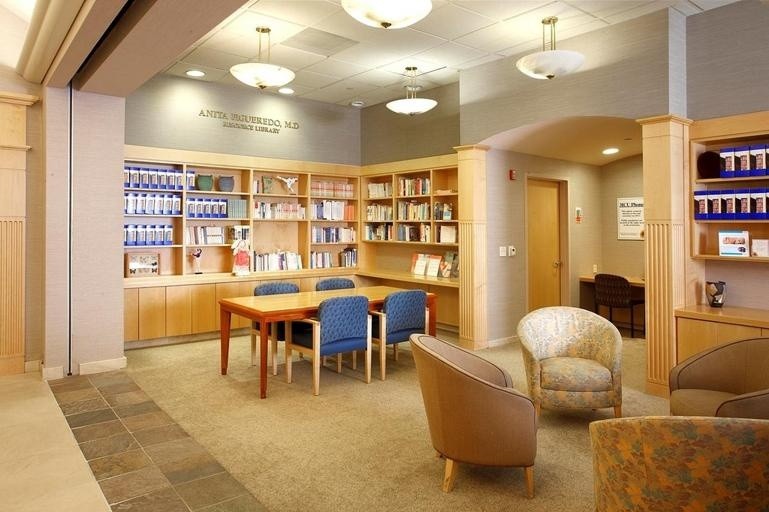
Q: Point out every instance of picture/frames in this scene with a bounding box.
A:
[126,252,161,278]
[617,196,644,242]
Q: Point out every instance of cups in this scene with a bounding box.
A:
[705,281,727,306]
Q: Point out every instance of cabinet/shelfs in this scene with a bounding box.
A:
[122,143,458,352]
[676,316,769,367]
[689,131,769,264]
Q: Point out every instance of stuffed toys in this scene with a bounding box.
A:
[229,238,251,277]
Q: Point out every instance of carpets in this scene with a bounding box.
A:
[0,372,112,512]
[125,333,669,511]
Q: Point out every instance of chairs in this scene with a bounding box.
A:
[669,336,769,418]
[250,281,312,376]
[516,304,623,419]
[352,289,430,380]
[408,333,538,499]
[316,277,355,290]
[589,415,769,512]
[594,274,645,338]
[284,296,372,396]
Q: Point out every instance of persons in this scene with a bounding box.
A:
[191,247,203,273]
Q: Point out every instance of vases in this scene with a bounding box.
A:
[197,174,234,192]
[706,280,726,308]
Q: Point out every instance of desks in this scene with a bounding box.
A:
[579,274,645,308]
[217,283,437,399]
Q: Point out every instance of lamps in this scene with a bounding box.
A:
[229,26,295,90]
[385,67,439,116]
[515,15,587,79]
[340,0,433,30]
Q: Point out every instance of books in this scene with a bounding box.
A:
[250,172,358,269]
[409,250,460,280]
[186,222,250,247]
[360,174,458,245]
[226,198,247,219]
